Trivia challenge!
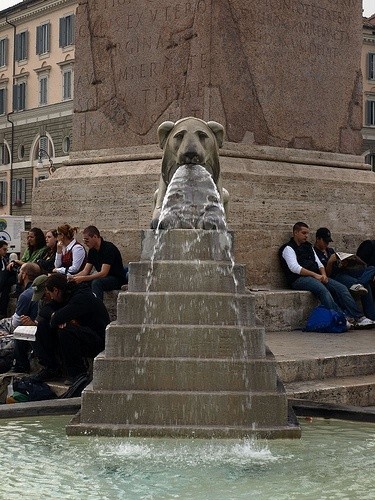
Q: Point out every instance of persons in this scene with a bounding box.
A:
[314,228,374,320]
[1,225,128,384]
[280,222,374,330]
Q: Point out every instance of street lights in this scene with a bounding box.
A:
[36,148,56,175]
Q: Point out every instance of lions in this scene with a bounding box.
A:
[150,117,230,229]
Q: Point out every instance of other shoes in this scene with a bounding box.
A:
[12,378,52,400]
[31,368,63,381]
[12,362,30,372]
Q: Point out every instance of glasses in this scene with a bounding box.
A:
[83,239,89,243]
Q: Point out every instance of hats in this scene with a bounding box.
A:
[30,275,48,301]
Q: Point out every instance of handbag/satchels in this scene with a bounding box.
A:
[0,317,16,373]
